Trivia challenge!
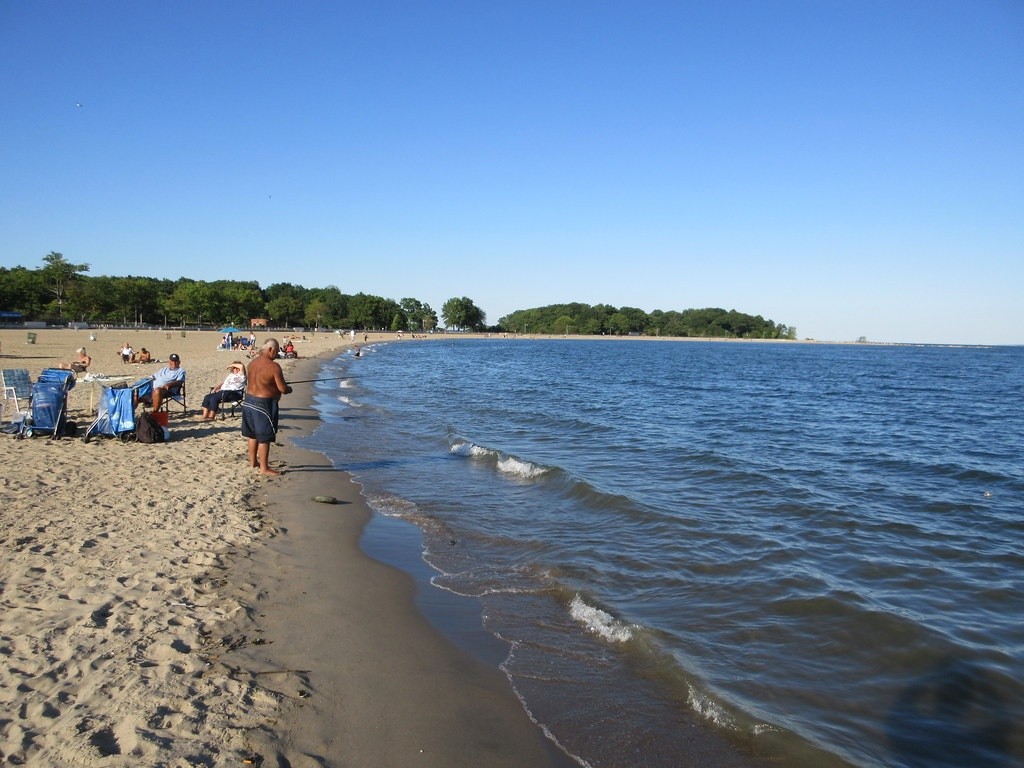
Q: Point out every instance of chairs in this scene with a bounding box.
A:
[70,357,91,380]
[210,386,245,421]
[1,367,36,415]
[142,376,186,415]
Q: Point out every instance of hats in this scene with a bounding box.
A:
[76,347,86,353]
[169,354,179,362]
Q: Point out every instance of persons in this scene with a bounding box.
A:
[199,361,247,421]
[412,334,427,339]
[278,337,297,359]
[120,343,139,365]
[134,353,186,412]
[139,348,150,364]
[339,331,344,339]
[397,332,404,339]
[364,335,369,344]
[355,345,360,356]
[242,338,293,476]
[222,331,256,350]
[59,348,90,373]
[350,329,355,342]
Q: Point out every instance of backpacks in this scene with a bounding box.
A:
[136,411,164,443]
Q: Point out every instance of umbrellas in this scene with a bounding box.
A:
[398,330,403,333]
[219,327,241,350]
